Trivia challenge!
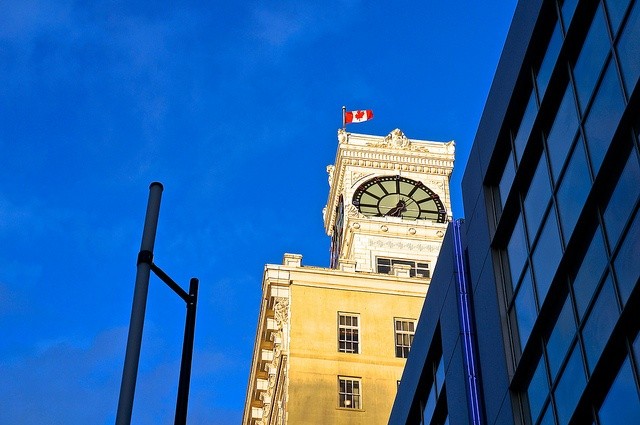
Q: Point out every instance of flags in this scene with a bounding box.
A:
[345,109,374,124]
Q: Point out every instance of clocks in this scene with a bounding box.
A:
[350,172,447,224]
